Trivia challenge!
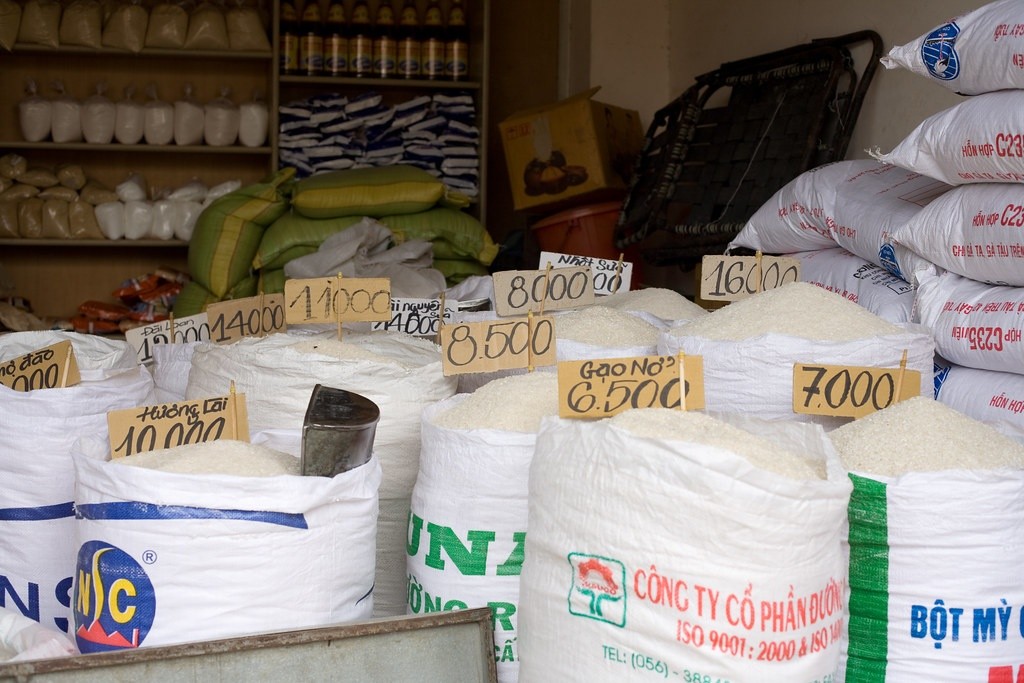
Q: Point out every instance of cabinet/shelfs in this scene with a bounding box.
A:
[0,0,491,316]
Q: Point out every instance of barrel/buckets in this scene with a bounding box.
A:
[532,201,649,291]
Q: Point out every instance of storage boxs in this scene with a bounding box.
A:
[495,86,647,213]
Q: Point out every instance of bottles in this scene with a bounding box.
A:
[372,0,398,78]
[280,0,298,75]
[398,0,421,79]
[444,0,471,83]
[299,0,325,75]
[324,0,347,75]
[422,0,446,81]
[347,0,373,77]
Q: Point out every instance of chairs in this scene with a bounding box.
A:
[614,28,884,265]
